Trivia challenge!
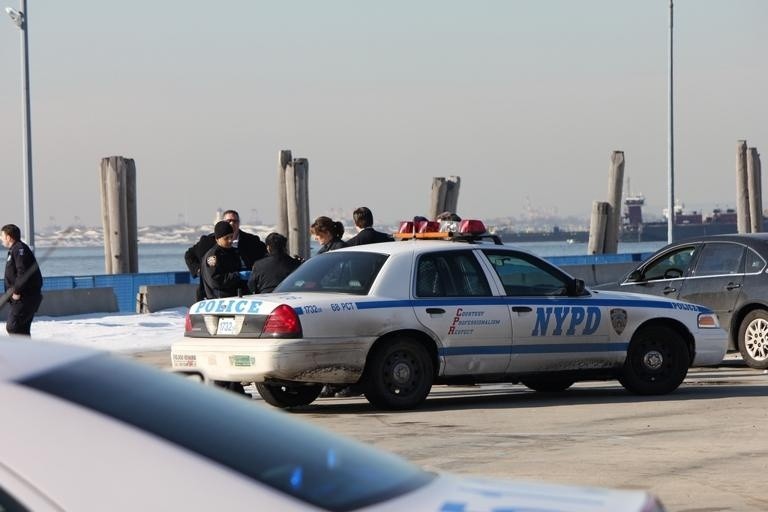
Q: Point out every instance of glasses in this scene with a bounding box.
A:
[226,218,238,223]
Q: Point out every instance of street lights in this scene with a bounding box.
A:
[6,5,37,275]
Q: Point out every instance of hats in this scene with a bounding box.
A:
[215,221,234,240]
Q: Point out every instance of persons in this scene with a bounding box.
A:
[0,224,44,336]
[200,221,252,398]
[246,233,301,294]
[185,210,269,279]
[309,216,348,255]
[345,206,395,246]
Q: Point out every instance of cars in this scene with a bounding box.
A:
[549,233,768,373]
[171,210,732,406]
[2,326,676,512]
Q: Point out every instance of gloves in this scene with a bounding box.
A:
[238,271,251,280]
